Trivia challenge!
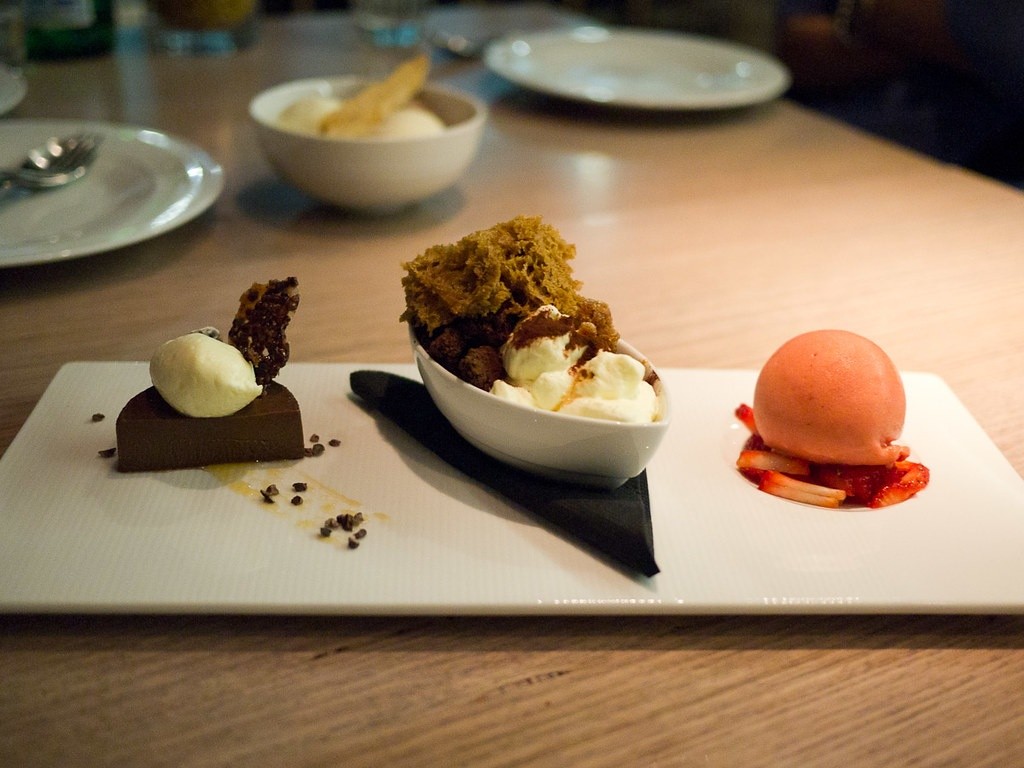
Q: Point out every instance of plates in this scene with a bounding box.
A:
[482,24,794,115]
[1,120,227,267]
[0,361,1023,616]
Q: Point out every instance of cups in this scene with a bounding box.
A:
[351,1,434,48]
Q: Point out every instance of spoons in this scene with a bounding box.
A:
[1,131,104,196]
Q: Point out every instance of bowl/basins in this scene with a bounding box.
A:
[246,72,487,216]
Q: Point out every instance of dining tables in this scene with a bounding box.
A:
[0,1,1024,768]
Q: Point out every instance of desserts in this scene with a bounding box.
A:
[736,329,930,508]
[116,277,306,473]
[400,211,661,426]
